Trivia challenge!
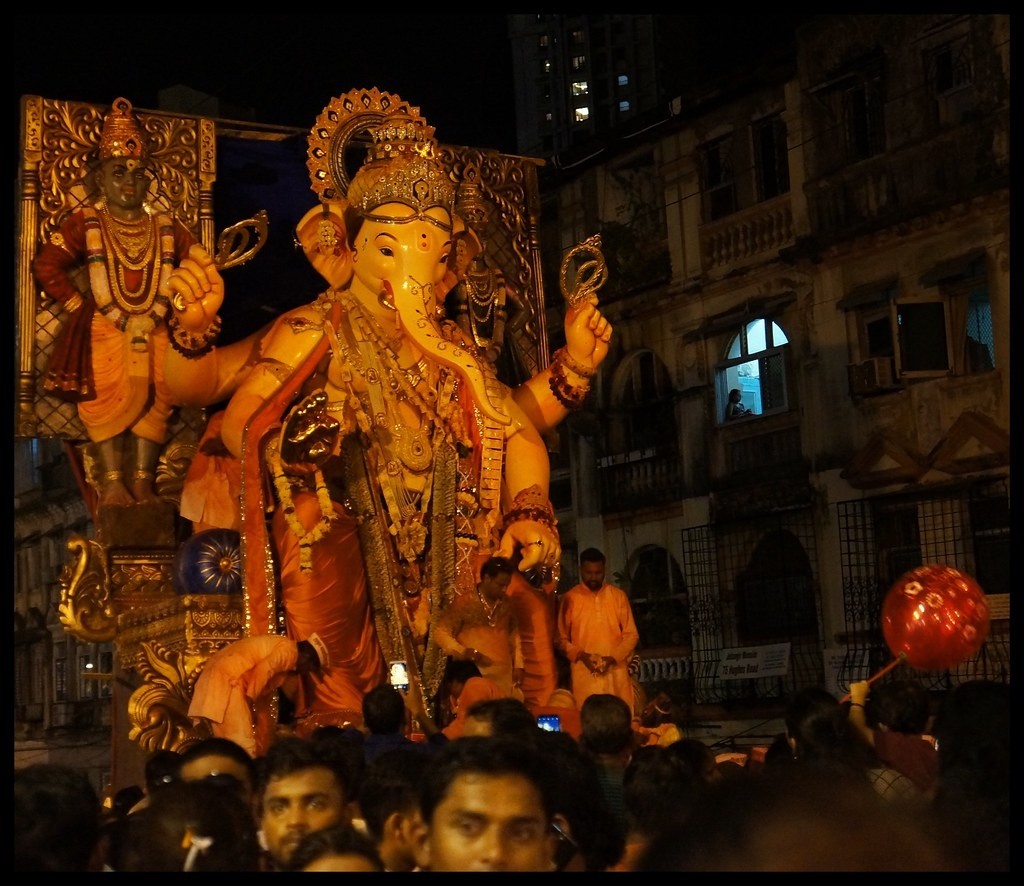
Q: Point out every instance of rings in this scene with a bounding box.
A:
[473,649,479,653]
[470,656,475,660]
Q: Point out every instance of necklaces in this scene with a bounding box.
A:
[480,597,498,627]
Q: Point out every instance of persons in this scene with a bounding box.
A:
[554,547,638,739]
[15,666,1012,871]
[432,163,532,380]
[186,631,329,763]
[30,98,198,516]
[433,556,526,724]
[721,385,755,420]
